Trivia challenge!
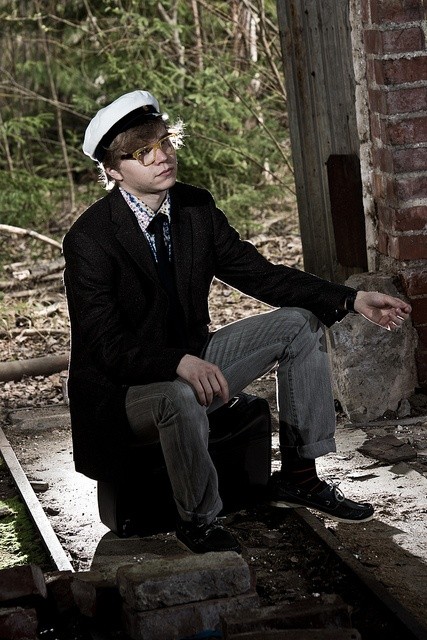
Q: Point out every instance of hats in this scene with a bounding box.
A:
[82,90,163,163]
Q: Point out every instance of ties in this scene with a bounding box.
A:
[146,212,170,281]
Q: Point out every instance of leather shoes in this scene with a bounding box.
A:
[269,470,374,524]
[173,520,242,558]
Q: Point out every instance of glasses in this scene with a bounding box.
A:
[116,133,179,166]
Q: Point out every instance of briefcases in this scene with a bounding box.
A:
[97,392,272,541]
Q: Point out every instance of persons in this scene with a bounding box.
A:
[62,89,413,554]
